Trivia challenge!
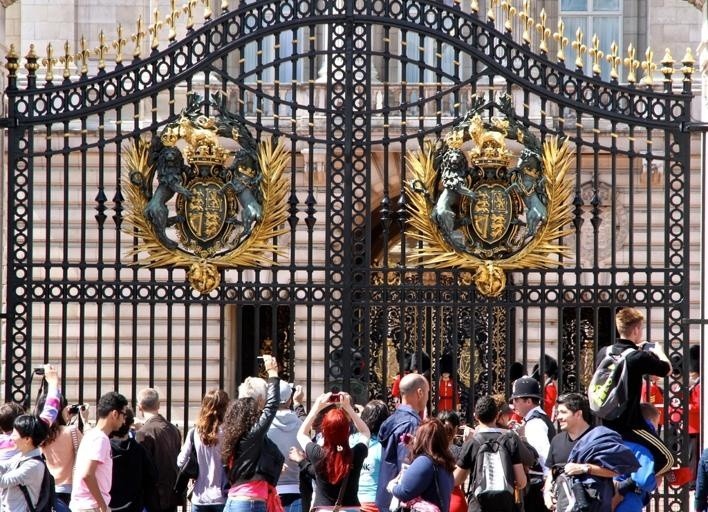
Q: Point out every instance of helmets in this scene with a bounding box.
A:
[509,378,544,401]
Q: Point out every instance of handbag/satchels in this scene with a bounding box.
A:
[401,496,441,512]
[180,445,198,480]
[549,463,602,512]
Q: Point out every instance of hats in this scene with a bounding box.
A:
[402,351,453,375]
[278,379,294,404]
[688,346,699,373]
[545,355,559,380]
[510,363,524,383]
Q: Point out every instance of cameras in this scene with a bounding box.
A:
[257,357,265,364]
[70,405,86,414]
[35,368,44,374]
[641,343,655,351]
[128,429,136,439]
[453,436,463,447]
[329,395,340,402]
[457,429,464,435]
[400,432,410,444]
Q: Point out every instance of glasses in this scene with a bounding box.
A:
[120,411,127,419]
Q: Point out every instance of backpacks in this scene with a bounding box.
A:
[587,344,637,421]
[472,432,523,511]
[16,454,56,511]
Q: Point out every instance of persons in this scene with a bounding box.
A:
[0,363,188,512]
[178,350,655,512]
[593,308,675,512]
[641,348,708,512]
